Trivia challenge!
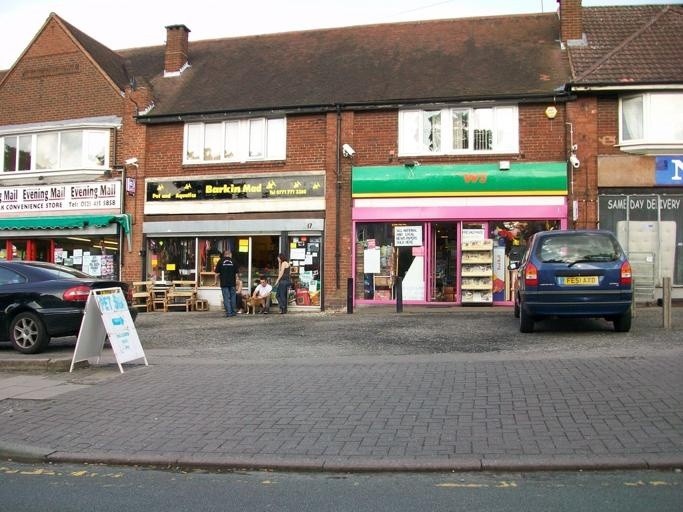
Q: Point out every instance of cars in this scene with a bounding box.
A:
[1,258,135,353]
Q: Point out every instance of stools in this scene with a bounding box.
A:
[195,299,209,311]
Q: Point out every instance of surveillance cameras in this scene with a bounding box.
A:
[124,158,137,166]
[569,155,580,169]
[342,144,356,156]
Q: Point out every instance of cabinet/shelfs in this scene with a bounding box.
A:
[460,239,494,307]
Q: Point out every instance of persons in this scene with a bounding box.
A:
[273,253,290,313]
[252,277,272,314]
[215,251,243,317]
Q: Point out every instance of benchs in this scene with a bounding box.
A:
[167,281,197,311]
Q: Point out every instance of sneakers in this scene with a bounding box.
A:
[221,308,287,317]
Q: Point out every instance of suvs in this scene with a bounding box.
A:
[507,228,634,333]
